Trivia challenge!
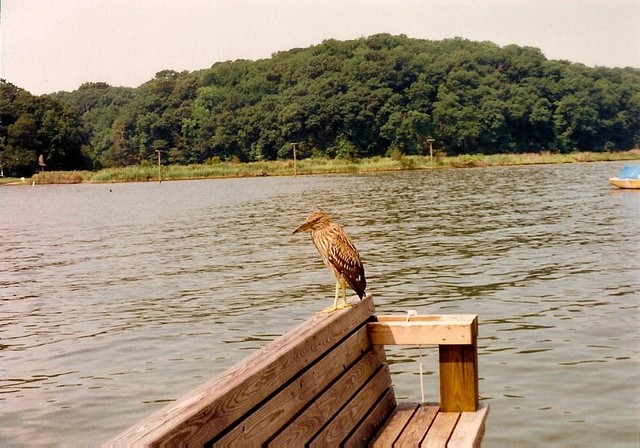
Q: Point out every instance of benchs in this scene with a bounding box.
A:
[99,291,491,447]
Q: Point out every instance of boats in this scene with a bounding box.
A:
[608,164,640,187]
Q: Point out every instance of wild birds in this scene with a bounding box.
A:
[293,212,367,315]
